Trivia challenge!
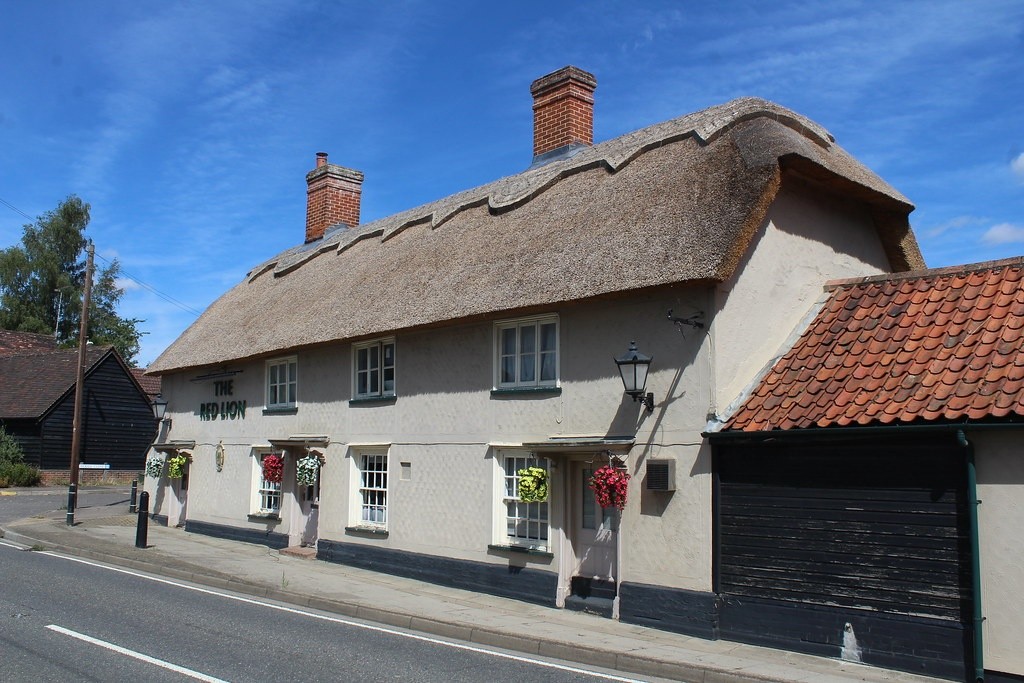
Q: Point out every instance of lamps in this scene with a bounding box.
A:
[150,394,172,429]
[613,339,654,413]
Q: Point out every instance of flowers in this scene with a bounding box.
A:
[146,458,165,479]
[168,455,187,479]
[518,467,549,504]
[295,453,322,487]
[587,465,631,511]
[262,454,285,484]
